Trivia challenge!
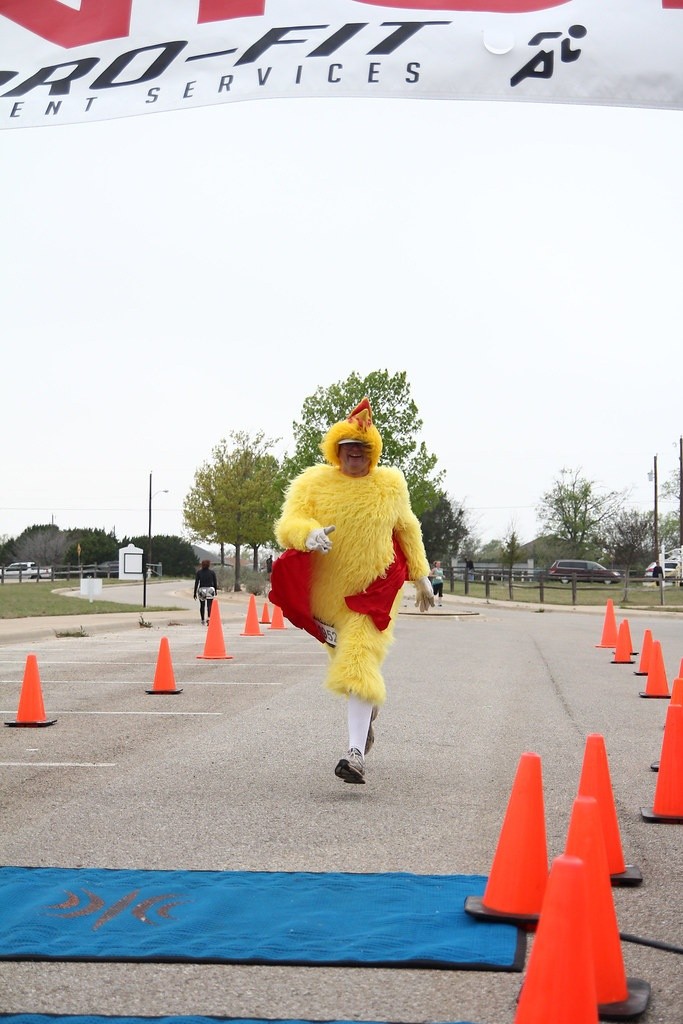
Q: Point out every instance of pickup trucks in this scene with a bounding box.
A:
[0,561,53,581]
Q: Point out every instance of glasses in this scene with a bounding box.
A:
[340,443,370,450]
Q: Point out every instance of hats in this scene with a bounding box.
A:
[338,440,362,444]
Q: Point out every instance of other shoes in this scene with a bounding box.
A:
[201,620,205,625]
[206,619,209,626]
[438,604,442,606]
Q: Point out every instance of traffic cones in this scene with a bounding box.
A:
[594,598,619,648]
[195,599,236,659]
[239,594,265,636]
[640,656,683,823]
[575,733,645,888]
[258,602,272,624]
[611,618,639,655]
[639,640,672,698]
[610,622,636,664]
[144,636,184,695]
[632,629,654,675]
[512,795,653,1023]
[267,604,288,630]
[462,753,551,923]
[4,654,58,727]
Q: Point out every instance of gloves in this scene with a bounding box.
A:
[414,576,435,612]
[305,525,335,554]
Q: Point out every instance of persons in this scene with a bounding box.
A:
[429,560,446,607]
[193,560,217,626]
[268,398,434,784]
[653,560,664,587]
[266,555,274,582]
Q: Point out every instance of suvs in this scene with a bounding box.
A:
[644,559,682,584]
[547,559,622,585]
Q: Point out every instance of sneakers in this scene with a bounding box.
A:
[335,747,366,784]
[365,705,379,755]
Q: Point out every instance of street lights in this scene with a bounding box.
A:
[144,471,170,608]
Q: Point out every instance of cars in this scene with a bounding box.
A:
[83,561,119,579]
